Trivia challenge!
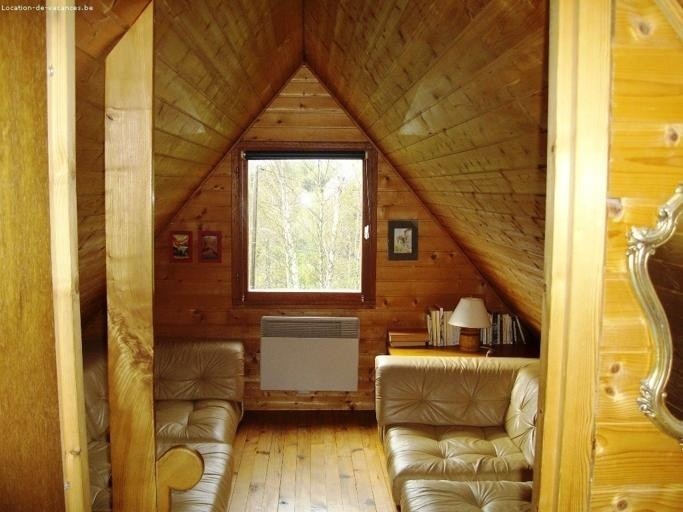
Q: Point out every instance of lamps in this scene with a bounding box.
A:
[448,297,492,351]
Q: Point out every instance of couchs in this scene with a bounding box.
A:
[83,338,245,512]
[374,355,539,512]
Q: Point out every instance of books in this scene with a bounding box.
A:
[390,341,426,347]
[425,304,527,346]
[387,330,426,341]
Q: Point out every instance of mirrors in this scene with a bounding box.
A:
[625,183,683,442]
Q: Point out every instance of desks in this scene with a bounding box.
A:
[387,345,517,357]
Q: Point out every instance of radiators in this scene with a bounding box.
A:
[258,316,360,393]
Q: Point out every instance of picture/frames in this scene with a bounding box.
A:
[169,230,193,264]
[388,221,419,260]
[197,231,221,263]
